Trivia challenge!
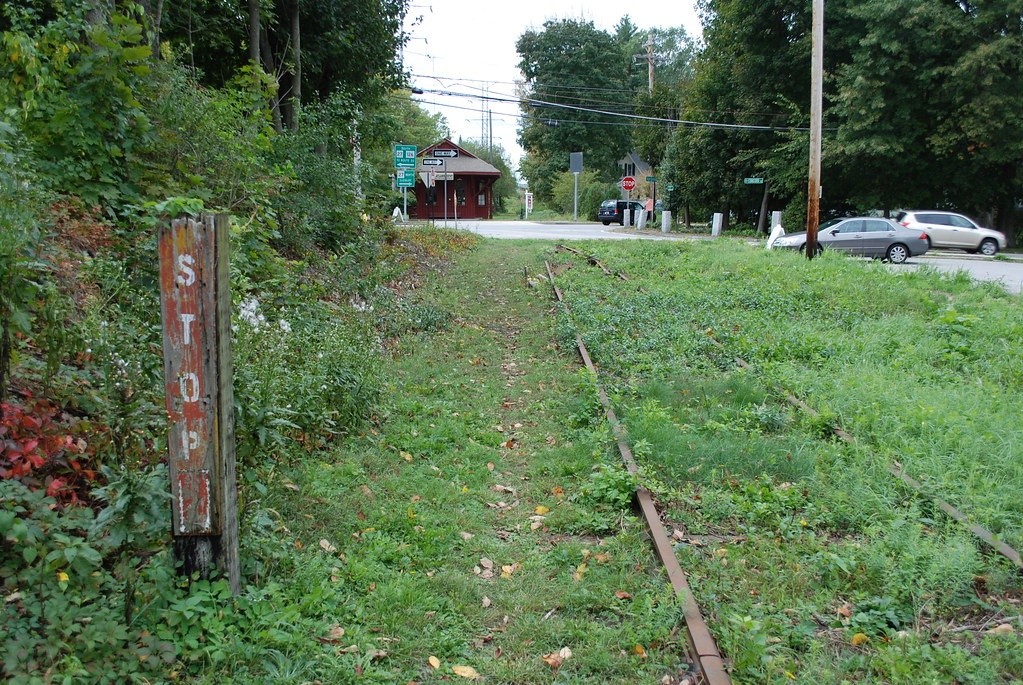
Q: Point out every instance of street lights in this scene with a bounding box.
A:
[485,80,497,158]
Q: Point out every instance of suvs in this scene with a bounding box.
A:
[598,199,656,226]
[899,210,1007,255]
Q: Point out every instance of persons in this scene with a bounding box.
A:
[646,196,656,223]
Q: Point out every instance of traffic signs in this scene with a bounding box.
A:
[422,159,443,166]
[433,149,459,159]
[397,170,415,187]
[395,145,416,169]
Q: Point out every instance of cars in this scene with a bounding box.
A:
[771,217,930,265]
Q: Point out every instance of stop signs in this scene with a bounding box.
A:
[622,177,635,191]
